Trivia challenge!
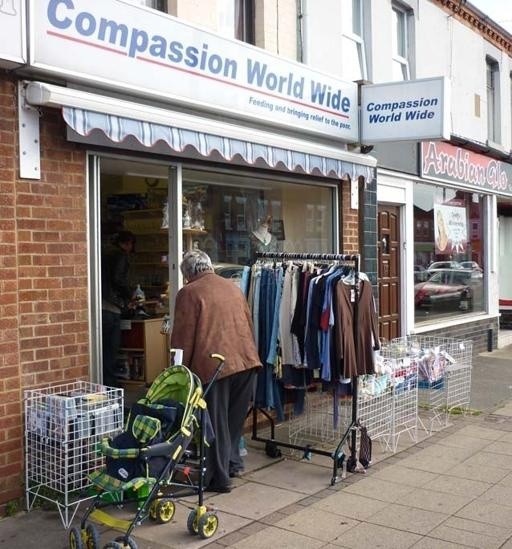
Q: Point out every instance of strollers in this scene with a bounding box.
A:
[67,348,225,549]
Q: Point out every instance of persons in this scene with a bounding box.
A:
[171,248,264,494]
[100,230,139,388]
[245,224,282,266]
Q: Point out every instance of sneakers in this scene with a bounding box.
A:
[205,485,232,494]
[229,468,246,478]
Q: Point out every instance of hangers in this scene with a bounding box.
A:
[255,250,364,285]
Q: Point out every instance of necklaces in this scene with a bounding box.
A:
[256,229,268,243]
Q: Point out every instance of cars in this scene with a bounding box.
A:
[414,269,483,312]
[460,260,483,272]
[415,264,427,282]
[426,261,464,271]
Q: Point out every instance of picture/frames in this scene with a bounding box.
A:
[269,220,286,240]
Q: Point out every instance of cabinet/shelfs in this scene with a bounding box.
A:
[113,207,208,387]
[22,378,125,531]
[287,333,474,457]
